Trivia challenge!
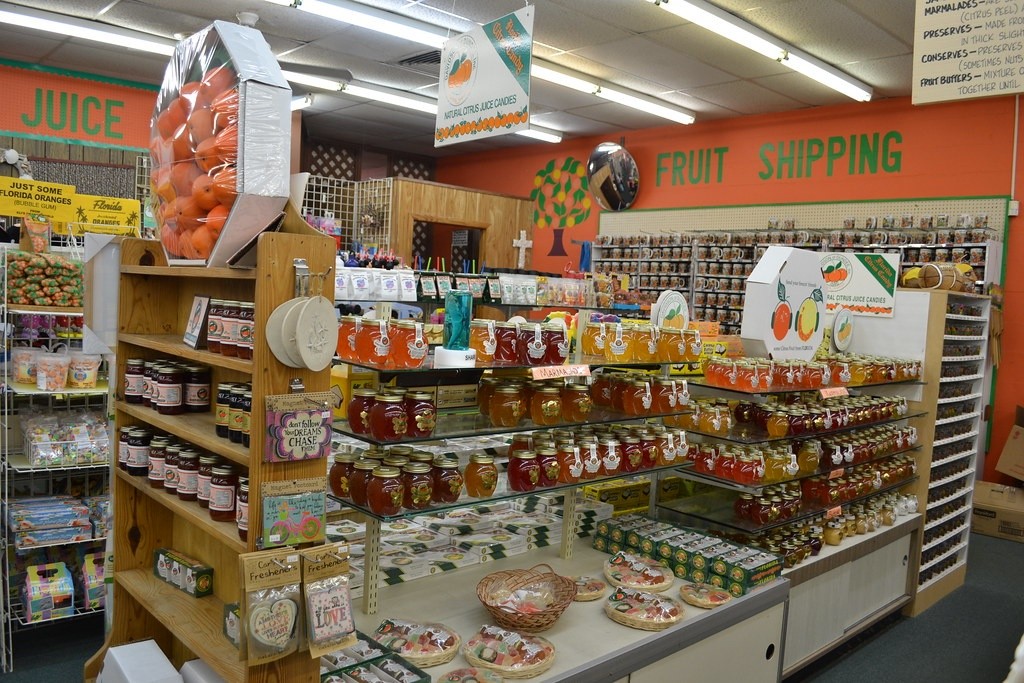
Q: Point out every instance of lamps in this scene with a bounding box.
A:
[0,2,872,146]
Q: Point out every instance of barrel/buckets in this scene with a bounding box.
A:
[67,347,104,388]
[36,342,71,392]
[10,346,46,384]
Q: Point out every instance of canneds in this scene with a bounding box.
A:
[118,424,249,542]
[124,357,251,449]
[329,316,922,569]
[207,298,255,361]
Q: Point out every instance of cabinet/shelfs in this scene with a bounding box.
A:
[326,345,927,537]
[0,191,335,683]
[844,289,992,621]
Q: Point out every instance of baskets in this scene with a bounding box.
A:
[477,563,577,633]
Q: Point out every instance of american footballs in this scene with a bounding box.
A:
[917,263,964,291]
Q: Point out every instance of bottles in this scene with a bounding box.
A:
[207,297,256,361]
[124,357,254,448]
[119,428,250,544]
[335,248,404,270]
[329,315,922,569]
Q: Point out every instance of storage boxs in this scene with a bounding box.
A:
[969,478,1024,543]
[740,242,827,365]
[995,424,1024,482]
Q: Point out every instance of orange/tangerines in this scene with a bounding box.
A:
[149,66,239,262]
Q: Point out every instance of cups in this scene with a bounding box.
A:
[441,288,473,350]
[595,215,998,335]
[413,266,597,309]
